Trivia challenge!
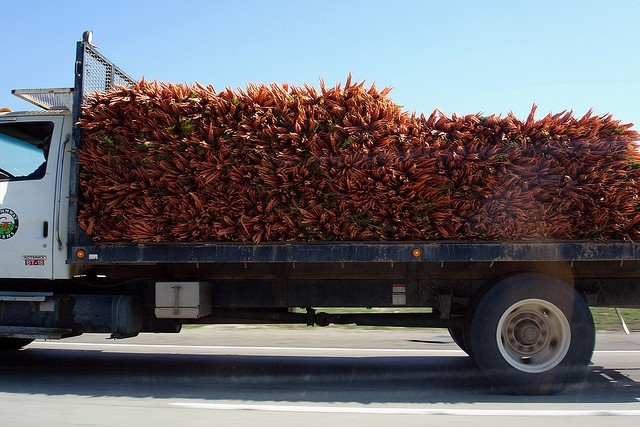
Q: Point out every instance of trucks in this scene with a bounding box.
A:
[0,29,640,395]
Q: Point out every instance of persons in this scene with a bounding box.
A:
[2,135,51,180]
